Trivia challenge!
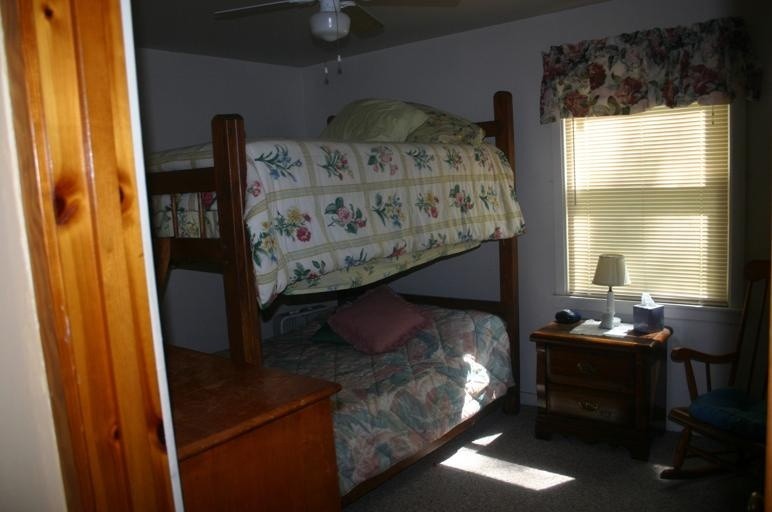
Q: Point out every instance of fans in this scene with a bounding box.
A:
[214,0,384,26]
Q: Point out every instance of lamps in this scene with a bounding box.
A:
[592,255,632,327]
[310,0,351,85]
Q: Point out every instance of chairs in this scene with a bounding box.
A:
[661,258,771,480]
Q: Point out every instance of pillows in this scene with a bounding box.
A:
[318,98,428,143]
[404,102,486,145]
[311,324,349,344]
[327,285,433,355]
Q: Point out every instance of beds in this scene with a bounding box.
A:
[148,91,520,512]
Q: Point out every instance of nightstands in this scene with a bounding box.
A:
[530,320,673,460]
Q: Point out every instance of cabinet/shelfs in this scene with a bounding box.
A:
[165,345,342,512]
